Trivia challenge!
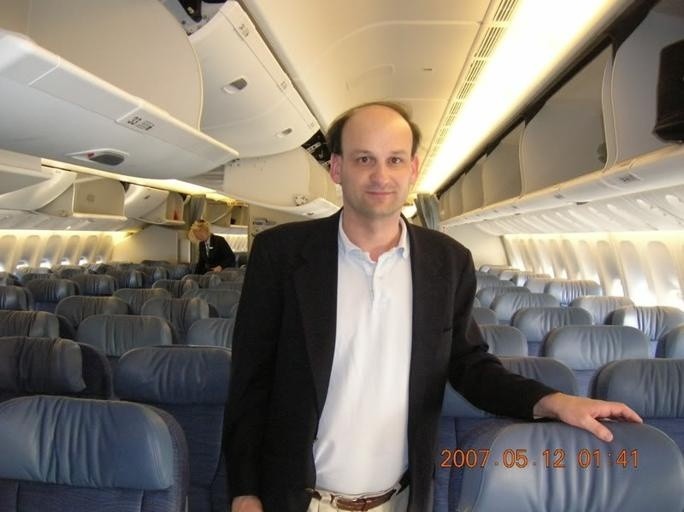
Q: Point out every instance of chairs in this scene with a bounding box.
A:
[426,263,683,511]
[1,259,244,512]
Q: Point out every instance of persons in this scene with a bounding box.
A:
[218,100,641,511]
[190,218,236,274]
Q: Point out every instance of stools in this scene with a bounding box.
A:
[315,492,394,511]
